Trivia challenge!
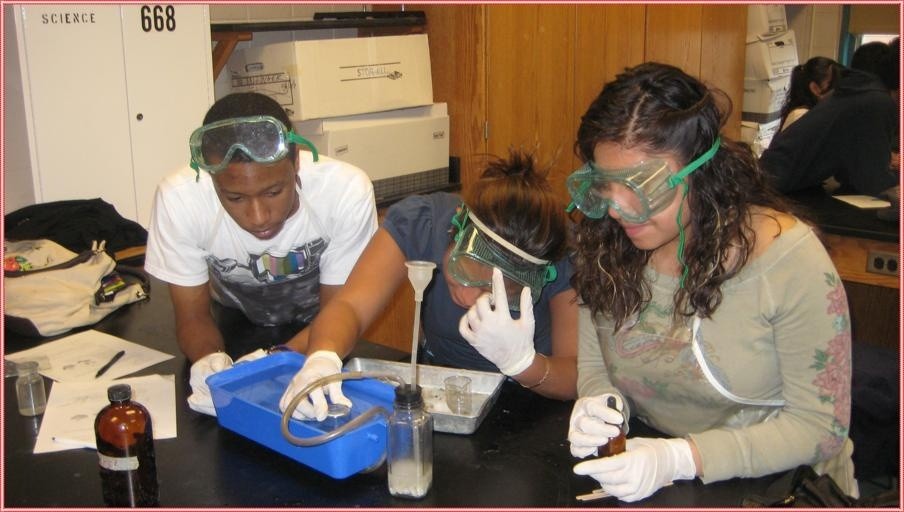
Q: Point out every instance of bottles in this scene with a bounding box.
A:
[93,382,158,508]
[11,361,48,417]
[599,396,627,459]
[386,383,434,500]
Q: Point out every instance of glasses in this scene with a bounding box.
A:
[449,223,548,312]
[189,115,289,173]
[565,158,678,224]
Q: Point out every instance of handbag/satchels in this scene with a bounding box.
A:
[5,198,146,265]
[5,238,151,340]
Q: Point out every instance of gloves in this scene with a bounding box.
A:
[573,436,697,503]
[567,393,624,459]
[278,350,352,422]
[458,268,536,378]
[187,347,267,418]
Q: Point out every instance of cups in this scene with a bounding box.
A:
[322,404,351,431]
[443,374,474,417]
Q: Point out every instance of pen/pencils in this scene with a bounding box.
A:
[96,351,125,377]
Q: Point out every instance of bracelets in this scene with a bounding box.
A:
[517,352,552,389]
[268,344,294,354]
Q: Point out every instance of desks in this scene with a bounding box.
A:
[4,251,819,506]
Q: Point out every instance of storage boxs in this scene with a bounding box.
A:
[286,102,450,202]
[739,3,802,161]
[214,30,435,126]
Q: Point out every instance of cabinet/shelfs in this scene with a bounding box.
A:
[642,4,747,153]
[380,3,645,229]
[4,4,216,233]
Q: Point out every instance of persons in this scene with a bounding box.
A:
[279,144,581,424]
[567,62,859,502]
[144,94,381,417]
[760,38,899,192]
